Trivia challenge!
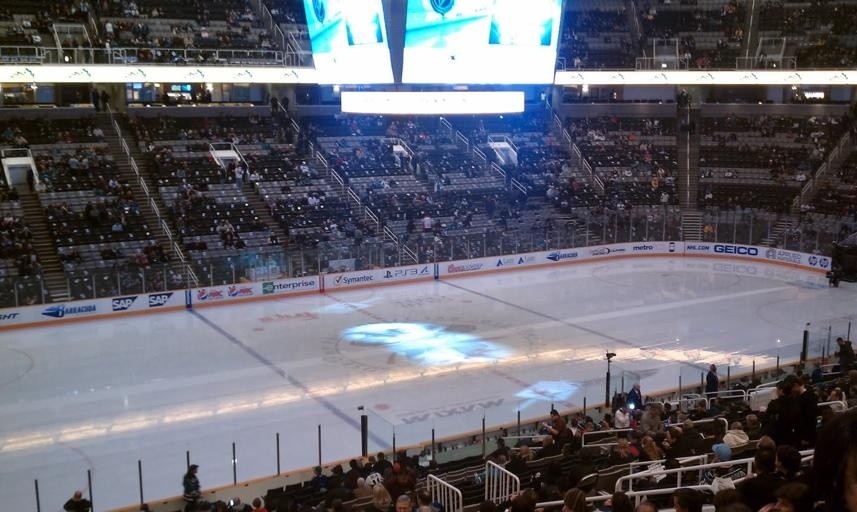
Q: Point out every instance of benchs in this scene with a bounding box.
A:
[40,2,557,71]
[23,3,313,66]
[22,84,829,307]
[265,365,847,511]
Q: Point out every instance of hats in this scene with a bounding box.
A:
[712,444,732,460]
